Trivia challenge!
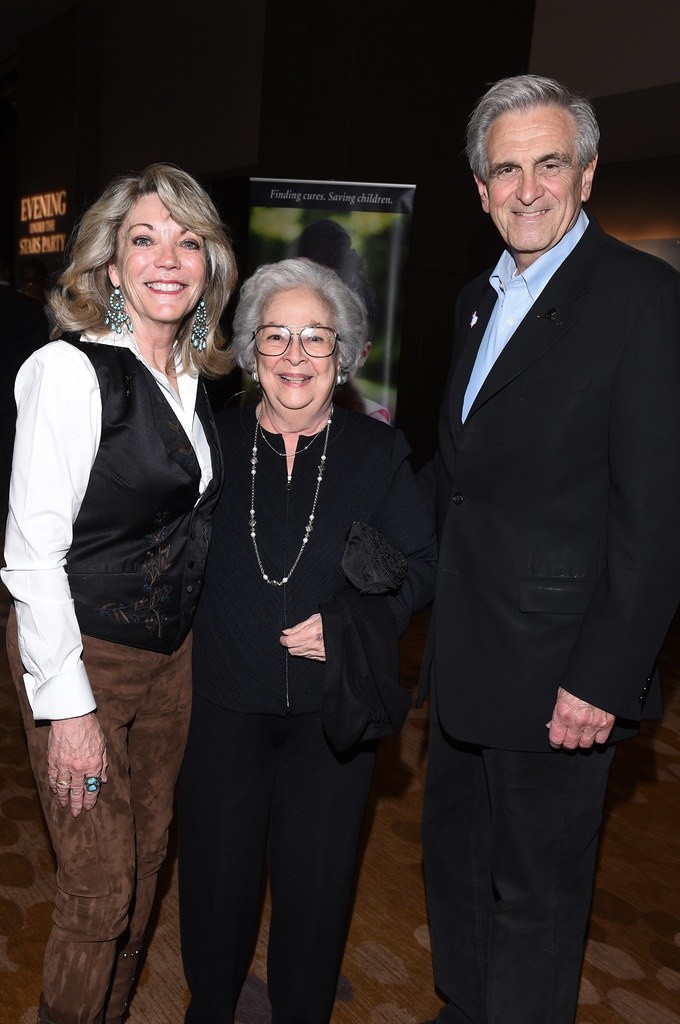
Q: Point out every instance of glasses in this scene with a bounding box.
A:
[252,325,341,358]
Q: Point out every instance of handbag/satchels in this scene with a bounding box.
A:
[342,522,407,594]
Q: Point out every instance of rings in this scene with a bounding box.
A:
[57,780,71,784]
[84,776,100,792]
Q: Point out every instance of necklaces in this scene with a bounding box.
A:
[250,404,333,586]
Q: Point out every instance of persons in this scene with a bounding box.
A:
[0,164,236,1024]
[420,76,680,1024]
[170,254,438,1023]
